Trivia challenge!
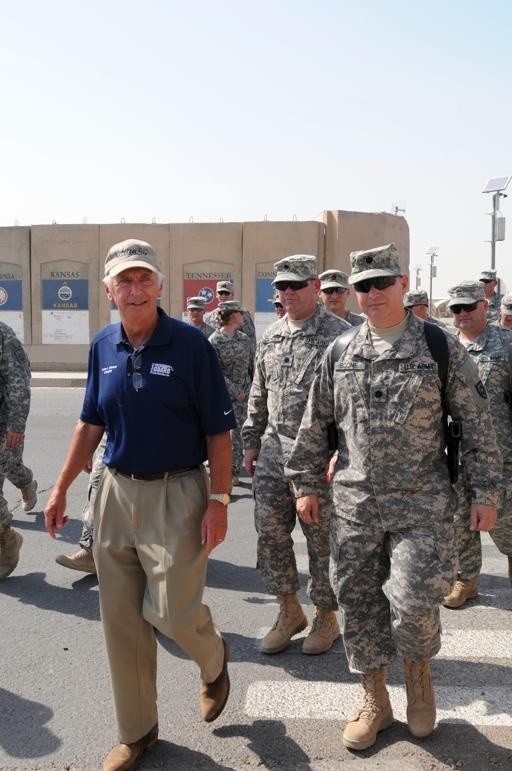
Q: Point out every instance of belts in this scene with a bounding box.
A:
[117,468,198,482]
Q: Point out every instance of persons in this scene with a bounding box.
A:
[55,430,108,575]
[283,242,503,753]
[44,239,237,771]
[441,282,511,608]
[0,319,38,577]
[189,249,512,490]
[241,255,352,655]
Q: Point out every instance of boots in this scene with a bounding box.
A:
[402,657,437,740]
[232,473,240,486]
[443,579,479,610]
[1,479,96,579]
[342,669,394,751]
[260,593,308,654]
[302,606,339,654]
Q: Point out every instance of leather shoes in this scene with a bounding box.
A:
[103,725,159,771]
[200,638,230,723]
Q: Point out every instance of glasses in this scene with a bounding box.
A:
[450,302,479,313]
[479,279,496,283]
[132,350,145,395]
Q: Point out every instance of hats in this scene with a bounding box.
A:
[403,290,428,307]
[105,240,160,278]
[272,243,409,307]
[447,281,485,307]
[478,270,496,280]
[501,296,512,317]
[187,281,242,310]
